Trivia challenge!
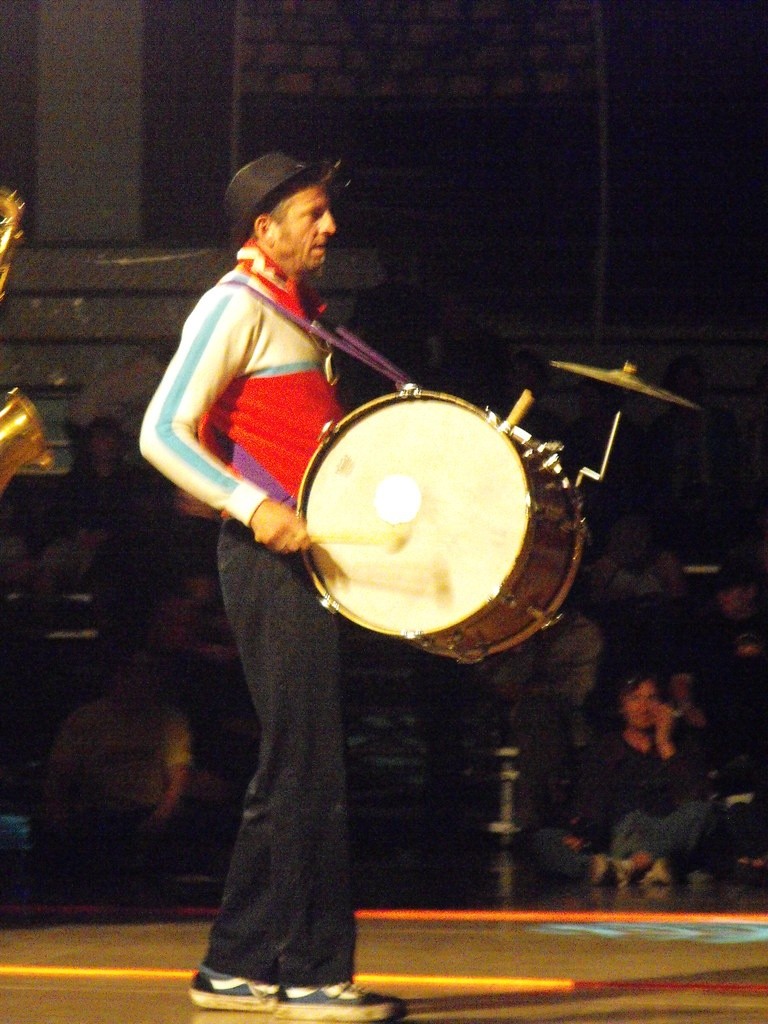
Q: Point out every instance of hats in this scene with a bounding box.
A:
[222,153,322,242]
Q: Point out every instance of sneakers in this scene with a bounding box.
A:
[188,969,281,1012]
[276,980,401,1022]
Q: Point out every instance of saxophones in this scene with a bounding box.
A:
[0,188,55,500]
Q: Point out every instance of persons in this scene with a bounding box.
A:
[0,415,259,845]
[340,245,768,891]
[138,149,405,1021]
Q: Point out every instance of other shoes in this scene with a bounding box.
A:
[591,854,634,888]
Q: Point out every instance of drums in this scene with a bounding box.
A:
[295,386,586,660]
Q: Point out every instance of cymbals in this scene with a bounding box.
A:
[554,362,702,410]
